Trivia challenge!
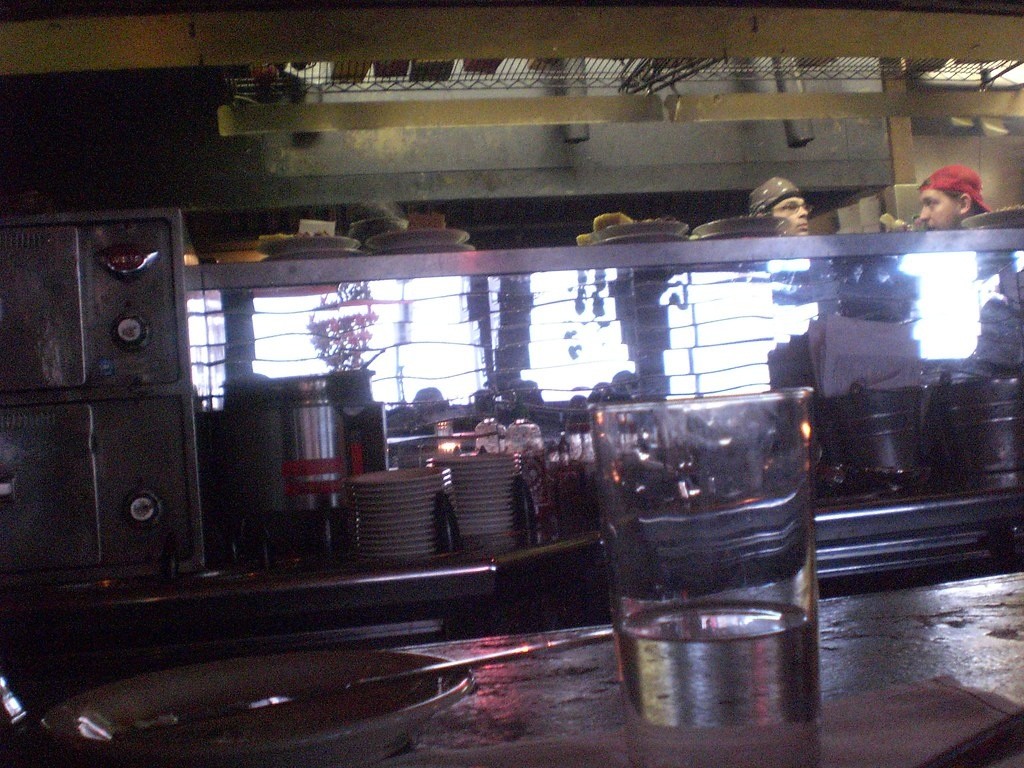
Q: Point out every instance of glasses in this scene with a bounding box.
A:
[769,203,815,215]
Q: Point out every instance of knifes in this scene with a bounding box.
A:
[132,627,614,731]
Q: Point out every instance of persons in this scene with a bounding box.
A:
[748,176,808,235]
[917,164,990,232]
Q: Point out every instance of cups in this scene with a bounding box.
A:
[590,384,822,731]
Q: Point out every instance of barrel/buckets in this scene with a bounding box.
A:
[835,375,1024,495]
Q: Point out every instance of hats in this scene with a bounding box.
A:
[918,163,992,213]
[748,176,800,214]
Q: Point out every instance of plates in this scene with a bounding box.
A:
[257,236,364,263]
[342,449,516,564]
[960,204,1024,230]
[692,217,784,239]
[590,222,689,246]
[42,650,476,768]
[366,228,476,256]
